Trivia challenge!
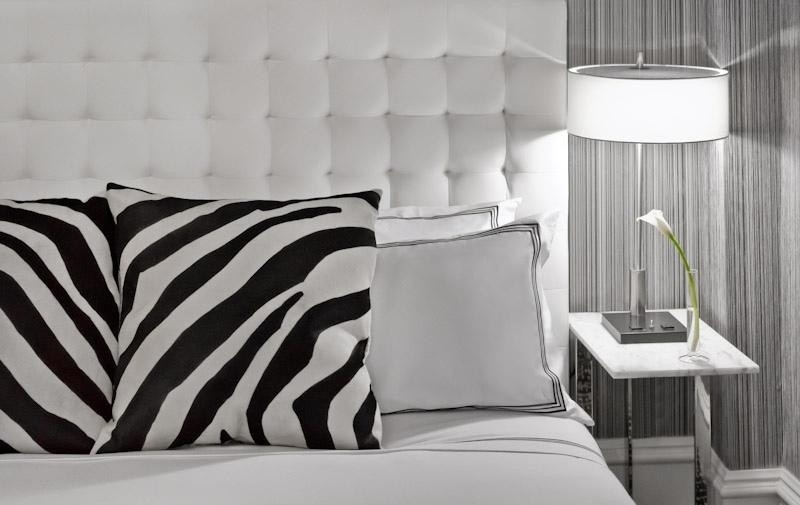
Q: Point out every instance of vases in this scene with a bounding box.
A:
[680,269,709,364]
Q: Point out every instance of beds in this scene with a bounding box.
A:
[0,0,642,505]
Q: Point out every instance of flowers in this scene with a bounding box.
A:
[635,207,699,351]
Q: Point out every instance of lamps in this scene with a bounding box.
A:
[567,51,728,345]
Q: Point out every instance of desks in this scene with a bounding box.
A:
[564,307,759,505]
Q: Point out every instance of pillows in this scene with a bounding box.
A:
[87,182,384,456]
[0,198,119,455]
[367,211,597,428]
[372,196,521,247]
